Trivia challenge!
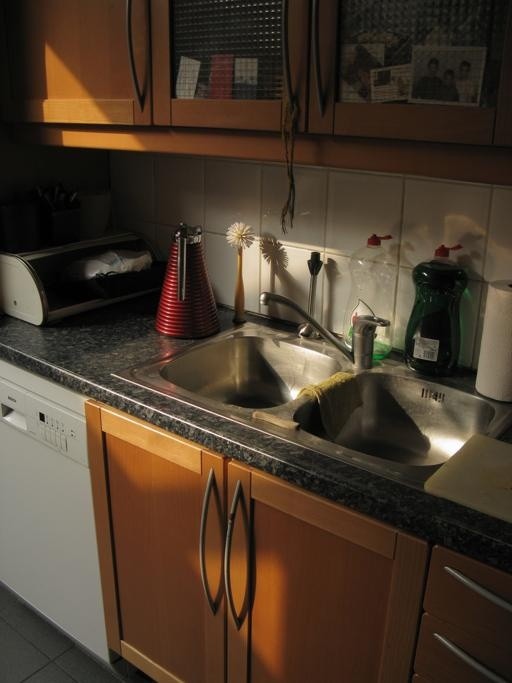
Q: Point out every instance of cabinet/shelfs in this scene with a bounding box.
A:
[4,1,152,157]
[412,540,510,680]
[152,1,509,185]
[87,401,429,682]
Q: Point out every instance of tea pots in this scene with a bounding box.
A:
[154,223,222,341]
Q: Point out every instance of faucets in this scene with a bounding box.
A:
[259,292,391,368]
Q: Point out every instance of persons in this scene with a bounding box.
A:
[454,59,480,102]
[438,68,458,100]
[413,56,443,98]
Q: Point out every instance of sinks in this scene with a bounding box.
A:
[292,372,511,499]
[111,322,343,418]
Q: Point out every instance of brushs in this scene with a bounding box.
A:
[226,221,254,323]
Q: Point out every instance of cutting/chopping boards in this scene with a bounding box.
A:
[420,432,512,526]
